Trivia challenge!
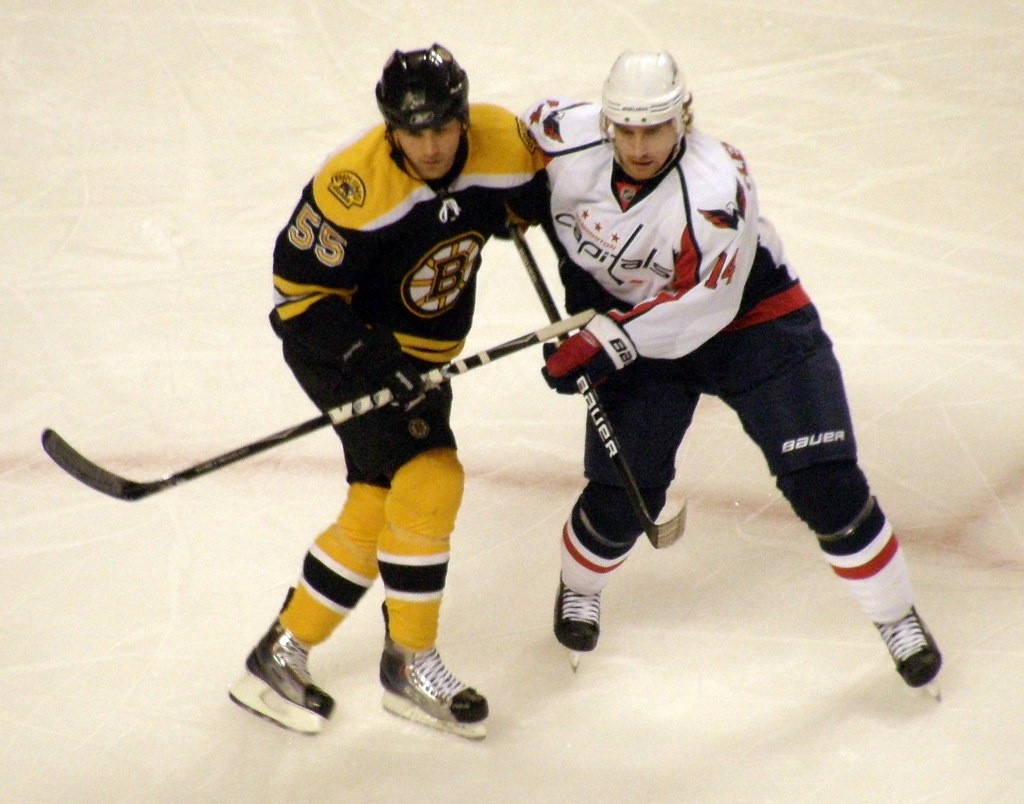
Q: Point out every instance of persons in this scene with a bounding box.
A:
[521,50,945,689]
[230,43,547,725]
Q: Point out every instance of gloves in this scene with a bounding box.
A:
[490,203,531,241]
[541,313,639,395]
[340,322,427,412]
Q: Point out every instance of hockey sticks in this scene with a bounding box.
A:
[38,304,600,504]
[511,223,691,551]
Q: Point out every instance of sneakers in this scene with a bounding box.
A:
[380,601,488,739]
[874,606,942,701]
[228,586,334,736]
[553,568,601,672]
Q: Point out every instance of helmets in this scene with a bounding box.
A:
[598,49,686,156]
[376,43,471,134]
